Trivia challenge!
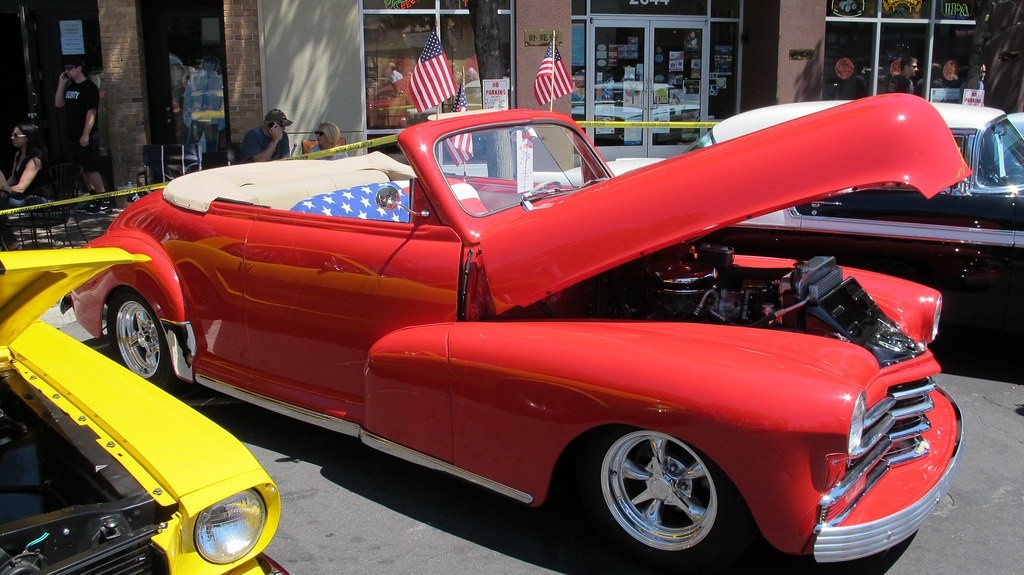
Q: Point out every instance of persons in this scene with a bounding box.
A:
[959,63,986,106]
[915,63,959,103]
[238,109,293,164]
[308,122,348,161]
[0,122,62,251]
[889,58,918,95]
[386,62,407,107]
[54,56,112,215]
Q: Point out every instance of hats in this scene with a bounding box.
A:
[265,109,293,126]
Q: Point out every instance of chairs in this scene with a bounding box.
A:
[141,142,202,185]
[5,160,90,250]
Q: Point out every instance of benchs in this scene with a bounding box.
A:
[288,176,488,223]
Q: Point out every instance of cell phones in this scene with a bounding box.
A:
[268,122,274,131]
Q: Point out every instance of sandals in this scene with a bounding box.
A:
[2,242,23,250]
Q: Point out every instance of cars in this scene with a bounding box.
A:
[406,78,485,121]
[0,245,291,575]
[531,99,1024,348]
[56,93,964,575]
[571,82,702,143]
[187,90,229,126]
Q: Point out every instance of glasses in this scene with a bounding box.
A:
[12,133,27,139]
[63,66,77,72]
[906,63,919,70]
[314,130,325,137]
[274,121,284,127]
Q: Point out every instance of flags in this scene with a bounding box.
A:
[409,25,460,113]
[442,80,474,166]
[533,40,575,105]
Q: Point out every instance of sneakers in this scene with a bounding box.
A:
[85,199,112,214]
